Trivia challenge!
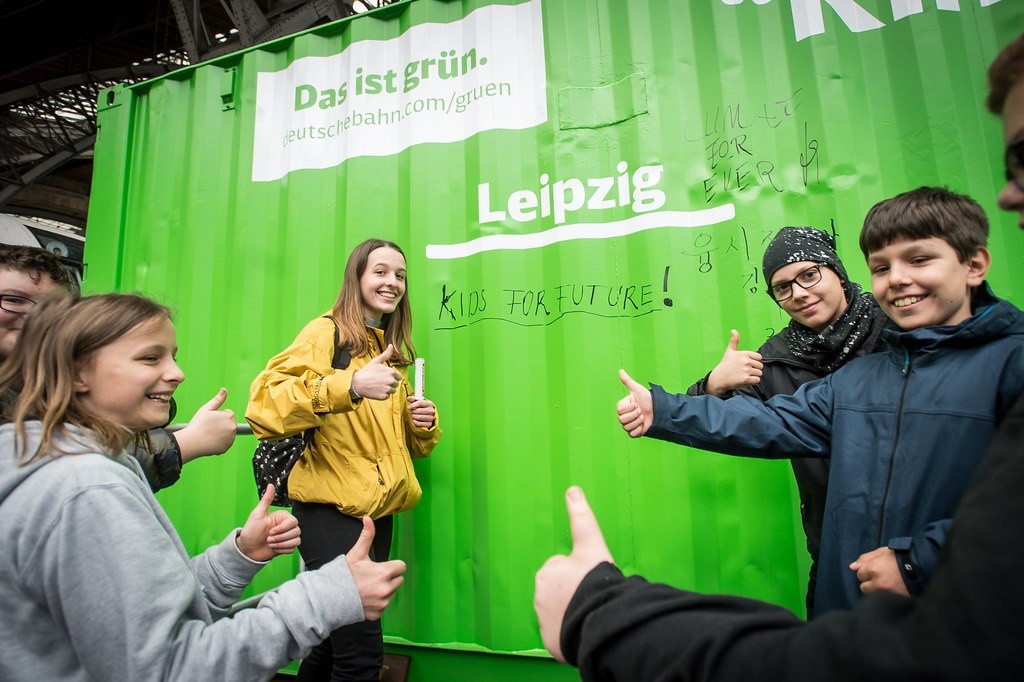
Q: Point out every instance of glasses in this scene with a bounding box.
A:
[1005,137,1024,192]
[764,261,827,302]
[0,294,36,314]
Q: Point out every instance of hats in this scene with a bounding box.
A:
[762,227,849,310]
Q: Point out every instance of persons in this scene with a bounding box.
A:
[616,185,1024,615]
[245,238,442,682]
[0,245,407,682]
[534,33,1024,682]
[687,226,885,621]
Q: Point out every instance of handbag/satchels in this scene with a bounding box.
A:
[251,316,351,508]
